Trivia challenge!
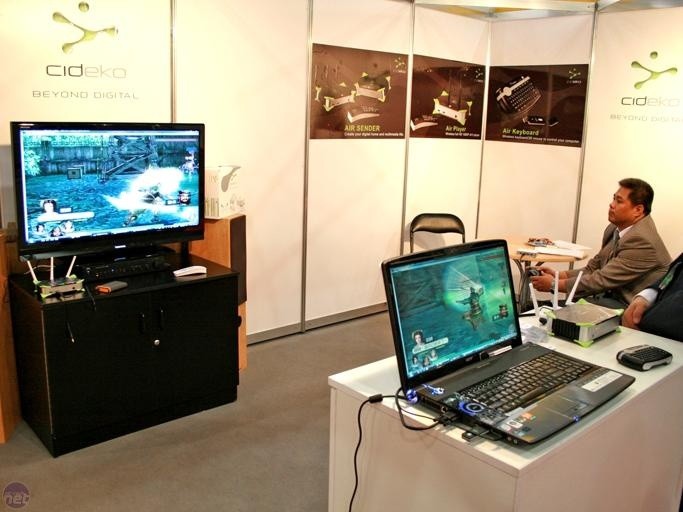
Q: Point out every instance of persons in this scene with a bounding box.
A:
[524,177,675,308]
[35,224,45,234]
[60,219,75,233]
[49,226,60,238]
[428,348,438,361]
[620,251,682,345]
[419,356,431,368]
[177,191,191,206]
[413,331,424,345]
[410,355,419,367]
[39,200,59,222]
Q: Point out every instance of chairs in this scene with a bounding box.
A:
[409,213,465,253]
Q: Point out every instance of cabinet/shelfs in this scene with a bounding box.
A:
[9,251,241,458]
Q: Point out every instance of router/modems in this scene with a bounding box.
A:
[25,256,86,297]
[529,270,622,348]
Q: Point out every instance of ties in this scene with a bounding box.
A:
[612,229,619,257]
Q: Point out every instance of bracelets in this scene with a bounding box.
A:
[551,278,555,293]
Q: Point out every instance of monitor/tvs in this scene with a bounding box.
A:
[10,122,204,282]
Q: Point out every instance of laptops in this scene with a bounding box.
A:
[381,239,635,445]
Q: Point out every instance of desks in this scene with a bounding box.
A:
[329,307,682,512]
[478,230,592,312]
